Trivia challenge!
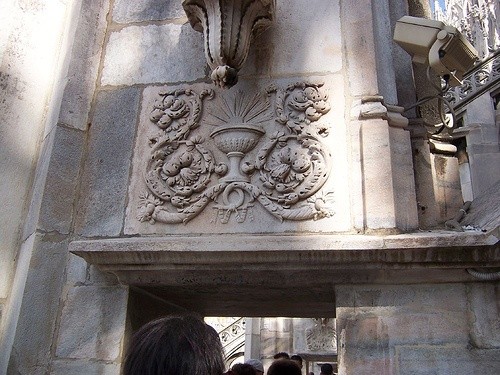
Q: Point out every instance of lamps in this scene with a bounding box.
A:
[393,15,481,141]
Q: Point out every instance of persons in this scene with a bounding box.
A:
[120,312,227,375]
[223,351,338,375]
